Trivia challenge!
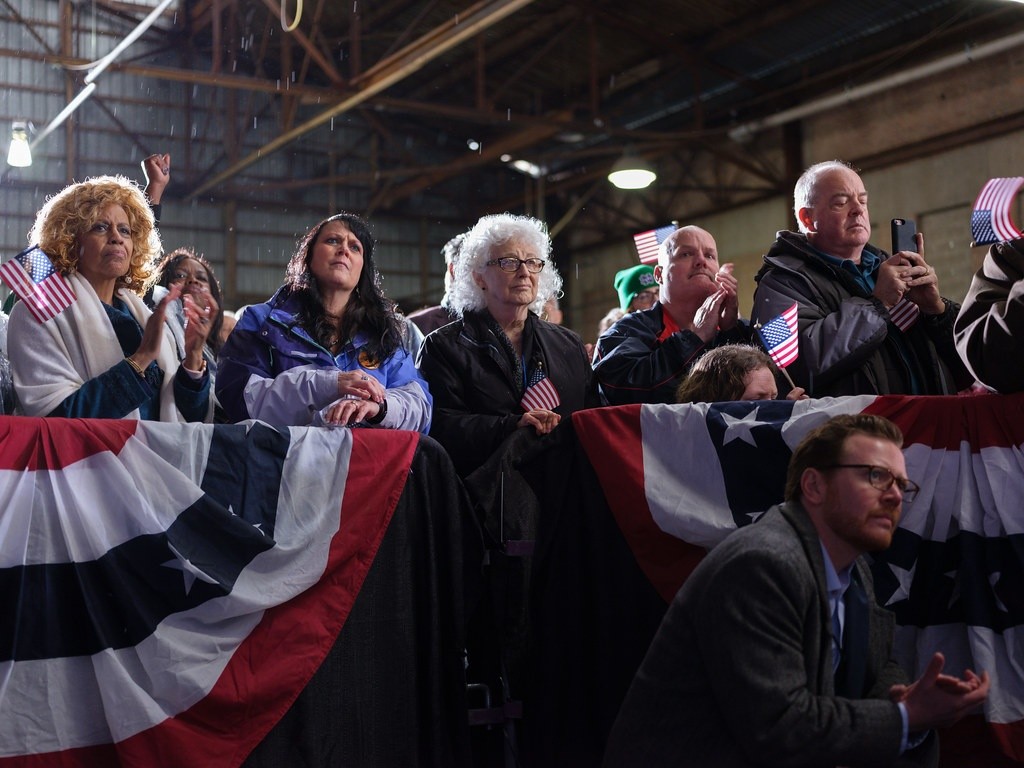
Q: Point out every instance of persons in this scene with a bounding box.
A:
[747,159,976,393]
[951,236,1024,395]
[0,151,810,435]
[603,413,991,768]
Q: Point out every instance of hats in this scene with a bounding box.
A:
[613,265,658,313]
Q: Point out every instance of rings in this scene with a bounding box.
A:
[363,376,370,381]
[921,264,930,277]
[351,399,358,410]
[200,317,209,324]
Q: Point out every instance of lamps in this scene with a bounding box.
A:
[6,117,32,167]
[605,146,656,189]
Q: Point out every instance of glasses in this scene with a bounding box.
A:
[485,255,546,274]
[825,463,922,506]
[635,289,660,305]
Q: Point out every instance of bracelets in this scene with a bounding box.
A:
[125,355,146,378]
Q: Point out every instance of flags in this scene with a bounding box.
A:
[760,300,799,369]
[521,370,561,414]
[633,226,680,264]
[0,247,79,325]
[970,176,1023,245]
[886,297,921,332]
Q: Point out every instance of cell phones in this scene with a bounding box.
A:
[891,217,918,267]
